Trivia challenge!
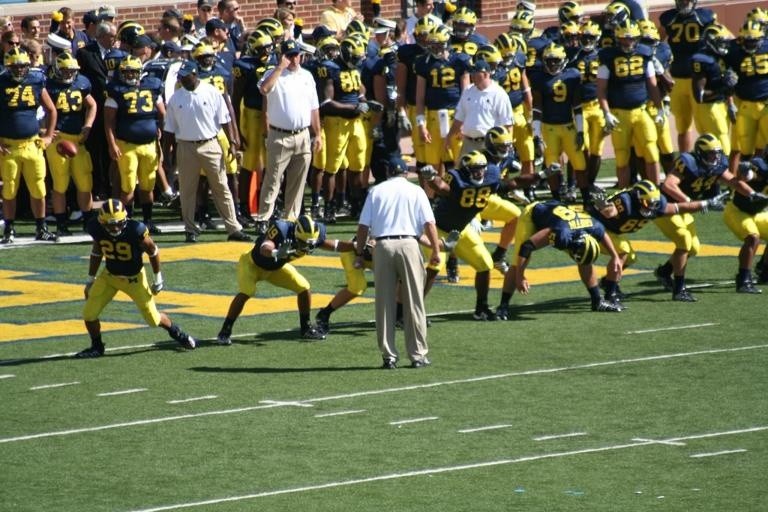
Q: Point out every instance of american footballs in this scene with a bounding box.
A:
[56,140,77,157]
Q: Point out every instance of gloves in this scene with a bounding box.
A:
[420,165,438,182]
[738,161,768,203]
[150,281,163,295]
[602,111,620,135]
[591,192,612,210]
[703,190,730,211]
[654,96,670,129]
[544,162,561,178]
[718,77,739,125]
[534,134,547,157]
[441,229,460,251]
[576,131,584,150]
[277,239,296,259]
[353,100,412,139]
[471,218,486,234]
[652,55,665,76]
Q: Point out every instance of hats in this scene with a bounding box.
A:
[389,157,408,174]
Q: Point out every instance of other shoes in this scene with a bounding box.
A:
[186,232,197,242]
[226,233,253,242]
[56,227,73,236]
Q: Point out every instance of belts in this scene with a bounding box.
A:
[193,135,218,144]
[375,235,417,240]
[269,123,309,134]
[465,137,484,141]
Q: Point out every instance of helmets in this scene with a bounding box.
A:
[97,198,127,234]
[4,17,370,84]
[568,234,601,265]
[414,1,660,75]
[458,125,513,188]
[700,7,768,57]
[694,132,723,173]
[294,214,319,245]
[631,179,661,218]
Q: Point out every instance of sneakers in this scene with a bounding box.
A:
[169,326,196,349]
[736,260,768,294]
[653,264,698,301]
[297,308,329,342]
[592,274,628,312]
[473,303,511,321]
[491,255,509,275]
[143,220,162,233]
[447,255,459,283]
[35,227,59,241]
[217,334,232,345]
[75,343,104,358]
[512,184,609,205]
[1,230,15,243]
[165,191,361,235]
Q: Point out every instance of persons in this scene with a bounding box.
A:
[0,0,768,370]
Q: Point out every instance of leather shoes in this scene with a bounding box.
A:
[383,361,432,369]
